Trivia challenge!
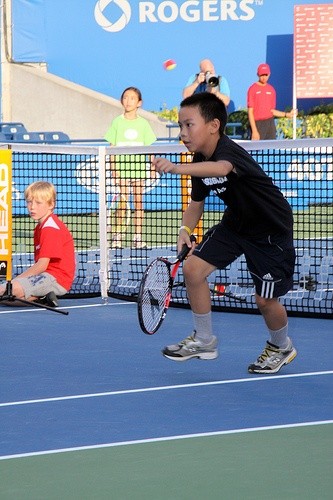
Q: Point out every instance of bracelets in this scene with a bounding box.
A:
[180,226,192,235]
[285,113,286,117]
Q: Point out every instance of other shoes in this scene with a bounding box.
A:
[111,234,121,248]
[134,240,146,249]
[38,290,58,307]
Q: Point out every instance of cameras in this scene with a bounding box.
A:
[204,71,220,87]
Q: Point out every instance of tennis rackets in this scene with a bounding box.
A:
[137,232,196,334]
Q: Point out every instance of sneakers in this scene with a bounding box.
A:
[162,330,218,361]
[247,336,297,373]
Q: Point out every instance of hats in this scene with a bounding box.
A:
[258,64,270,75]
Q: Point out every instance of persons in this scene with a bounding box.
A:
[153,92,297,374]
[183,58,230,106]
[247,64,298,140]
[104,87,157,249]
[0,181,76,306]
